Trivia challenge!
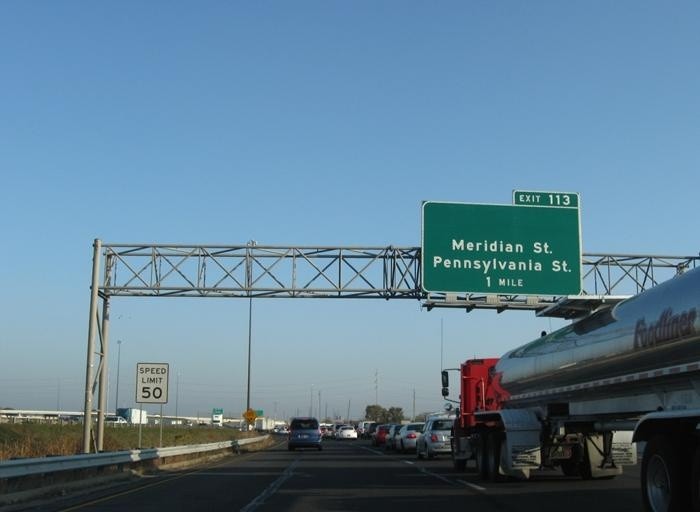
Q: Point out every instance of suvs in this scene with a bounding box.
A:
[414,417,458,461]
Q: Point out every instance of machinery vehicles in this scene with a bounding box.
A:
[441,265,700,512]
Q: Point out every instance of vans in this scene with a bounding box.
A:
[286,416,326,451]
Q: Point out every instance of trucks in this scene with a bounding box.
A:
[116,407,148,427]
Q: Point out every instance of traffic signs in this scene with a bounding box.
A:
[136,363,170,405]
[420,200,583,298]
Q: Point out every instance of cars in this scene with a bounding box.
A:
[103,415,127,427]
[383,424,405,452]
[370,424,396,449]
[271,421,386,441]
[395,422,426,455]
[234,425,244,432]
[183,419,222,428]
[57,415,79,425]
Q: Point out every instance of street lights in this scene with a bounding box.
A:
[115,340,122,416]
[175,368,183,424]
